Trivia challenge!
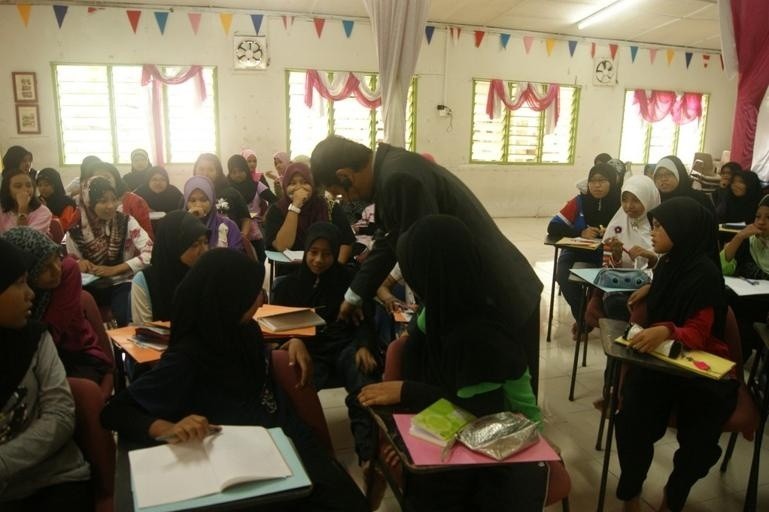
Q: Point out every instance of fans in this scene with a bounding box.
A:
[233,35,267,71]
[592,56,618,86]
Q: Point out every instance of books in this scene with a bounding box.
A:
[614,334,738,382]
[148,210,165,221]
[554,235,603,250]
[280,248,306,262]
[255,303,327,332]
[408,397,476,448]
[126,425,292,509]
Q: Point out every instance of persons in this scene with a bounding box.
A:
[265,152,292,200]
[264,164,358,265]
[0,168,52,240]
[547,163,618,340]
[377,261,417,312]
[720,195,769,280]
[2,238,92,512]
[355,215,560,470]
[272,222,384,460]
[616,197,740,510]
[713,161,743,204]
[2,145,33,172]
[86,163,154,241]
[603,176,662,270]
[183,174,247,256]
[652,155,713,209]
[67,178,152,327]
[65,155,102,196]
[119,148,154,191]
[36,167,76,232]
[308,136,544,407]
[99,246,315,508]
[130,209,213,372]
[240,148,270,187]
[3,227,113,387]
[226,155,279,214]
[192,153,252,240]
[716,170,767,223]
[134,165,184,214]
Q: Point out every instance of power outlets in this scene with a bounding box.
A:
[440,108,447,117]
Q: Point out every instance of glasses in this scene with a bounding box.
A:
[654,171,673,179]
[589,176,607,184]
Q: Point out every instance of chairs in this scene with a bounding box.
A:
[596,300,768,512]
[268,349,334,459]
[62,377,115,512]
[365,337,572,512]
[80,290,116,401]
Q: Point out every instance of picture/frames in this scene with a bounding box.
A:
[15,104,43,136]
[12,71,38,102]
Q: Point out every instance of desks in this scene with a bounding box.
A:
[595,316,736,511]
[360,397,564,510]
[567,261,641,402]
[115,427,374,512]
[543,232,603,343]
[262,249,304,305]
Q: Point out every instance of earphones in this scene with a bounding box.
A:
[341,178,350,189]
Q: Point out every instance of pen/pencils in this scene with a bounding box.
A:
[153,427,219,442]
[622,247,633,258]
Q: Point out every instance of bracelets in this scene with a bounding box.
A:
[18,212,28,219]
[289,203,301,215]
